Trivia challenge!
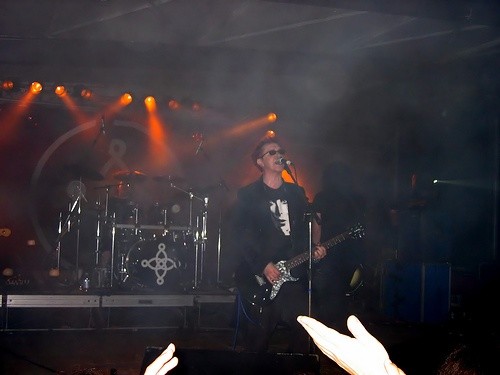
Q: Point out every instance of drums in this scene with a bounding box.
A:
[126,236,189,290]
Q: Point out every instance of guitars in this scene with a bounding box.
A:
[247,220,369,313]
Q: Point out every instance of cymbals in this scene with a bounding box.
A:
[61,162,105,181]
[112,169,147,183]
[152,174,186,184]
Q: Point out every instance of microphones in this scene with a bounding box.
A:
[278,158,294,166]
[100,116,106,136]
[195,139,205,154]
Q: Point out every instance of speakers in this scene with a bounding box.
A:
[139,346,320,375]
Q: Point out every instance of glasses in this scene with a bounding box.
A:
[260,149,286,158]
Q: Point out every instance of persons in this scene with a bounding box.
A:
[142,343,179,375]
[297,315,406,375]
[437,345,500,375]
[220,139,327,352]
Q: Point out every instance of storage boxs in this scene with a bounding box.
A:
[107,306,187,329]
[4,307,97,331]
[382,261,451,323]
[197,302,236,331]
